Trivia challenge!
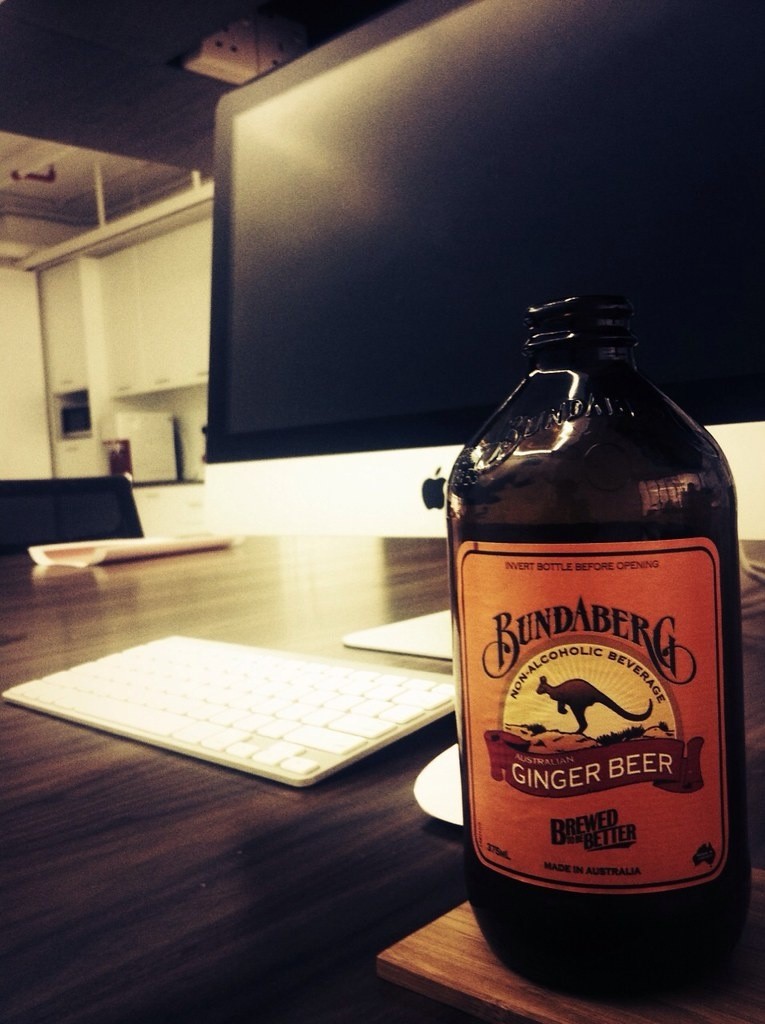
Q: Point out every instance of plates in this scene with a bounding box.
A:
[412,743,465,827]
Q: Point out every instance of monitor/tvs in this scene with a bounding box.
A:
[202,0,765,662]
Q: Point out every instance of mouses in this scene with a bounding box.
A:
[412,744,464,826]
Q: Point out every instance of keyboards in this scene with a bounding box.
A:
[1,636,458,786]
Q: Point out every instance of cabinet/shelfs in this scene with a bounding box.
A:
[36,216,213,538]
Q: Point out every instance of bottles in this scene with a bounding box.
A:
[446,293,752,1004]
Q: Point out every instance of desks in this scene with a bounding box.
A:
[0,536,765,1024]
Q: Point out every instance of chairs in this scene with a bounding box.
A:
[1,473,145,553]
